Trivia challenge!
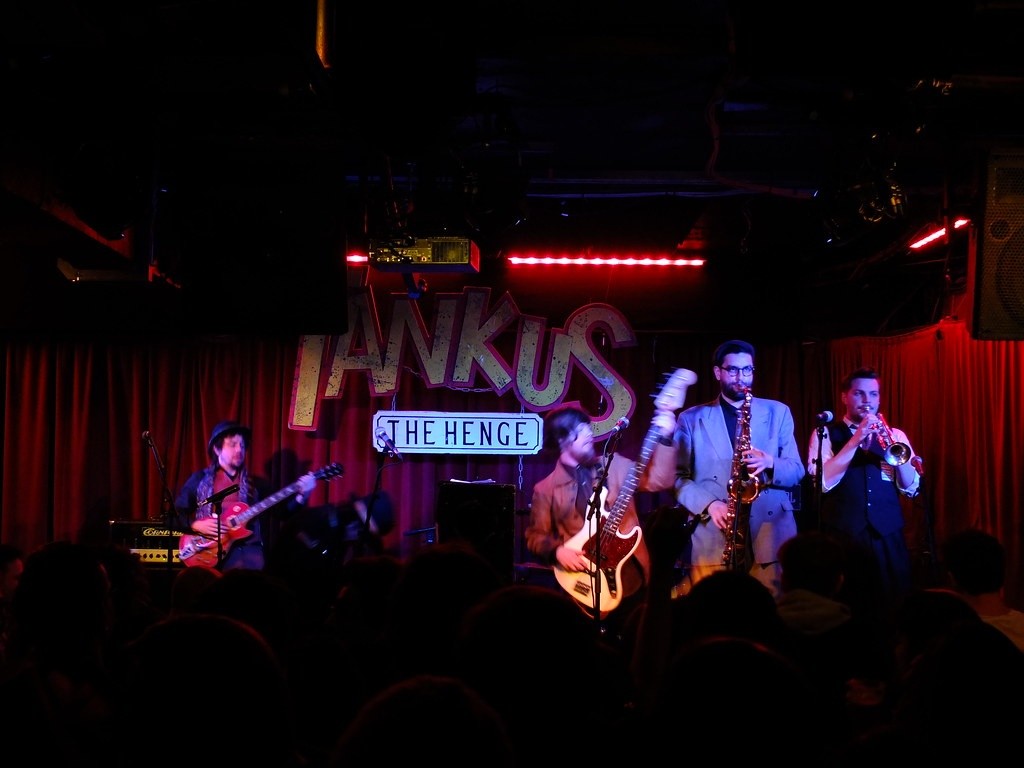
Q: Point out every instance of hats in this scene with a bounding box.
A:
[208,421,251,459]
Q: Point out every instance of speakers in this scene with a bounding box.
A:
[437,480,516,587]
[174,160,349,340]
[971,146,1024,341]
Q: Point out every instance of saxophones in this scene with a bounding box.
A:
[724,387,761,572]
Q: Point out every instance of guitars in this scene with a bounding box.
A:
[553,368,698,610]
[179,461,344,569]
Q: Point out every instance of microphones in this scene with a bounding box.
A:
[381,434,403,461]
[403,527,436,536]
[817,410,833,422]
[142,431,150,439]
[739,462,752,487]
[609,419,628,441]
[201,485,240,505]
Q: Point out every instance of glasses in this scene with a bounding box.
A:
[721,366,755,377]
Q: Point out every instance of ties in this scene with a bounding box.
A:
[851,424,869,452]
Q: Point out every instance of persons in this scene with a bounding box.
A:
[676,341,807,598]
[524,405,679,605]
[159,419,317,573]
[801,366,924,591]
[0,525,1024,768]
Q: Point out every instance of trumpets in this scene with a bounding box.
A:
[866,406,912,468]
